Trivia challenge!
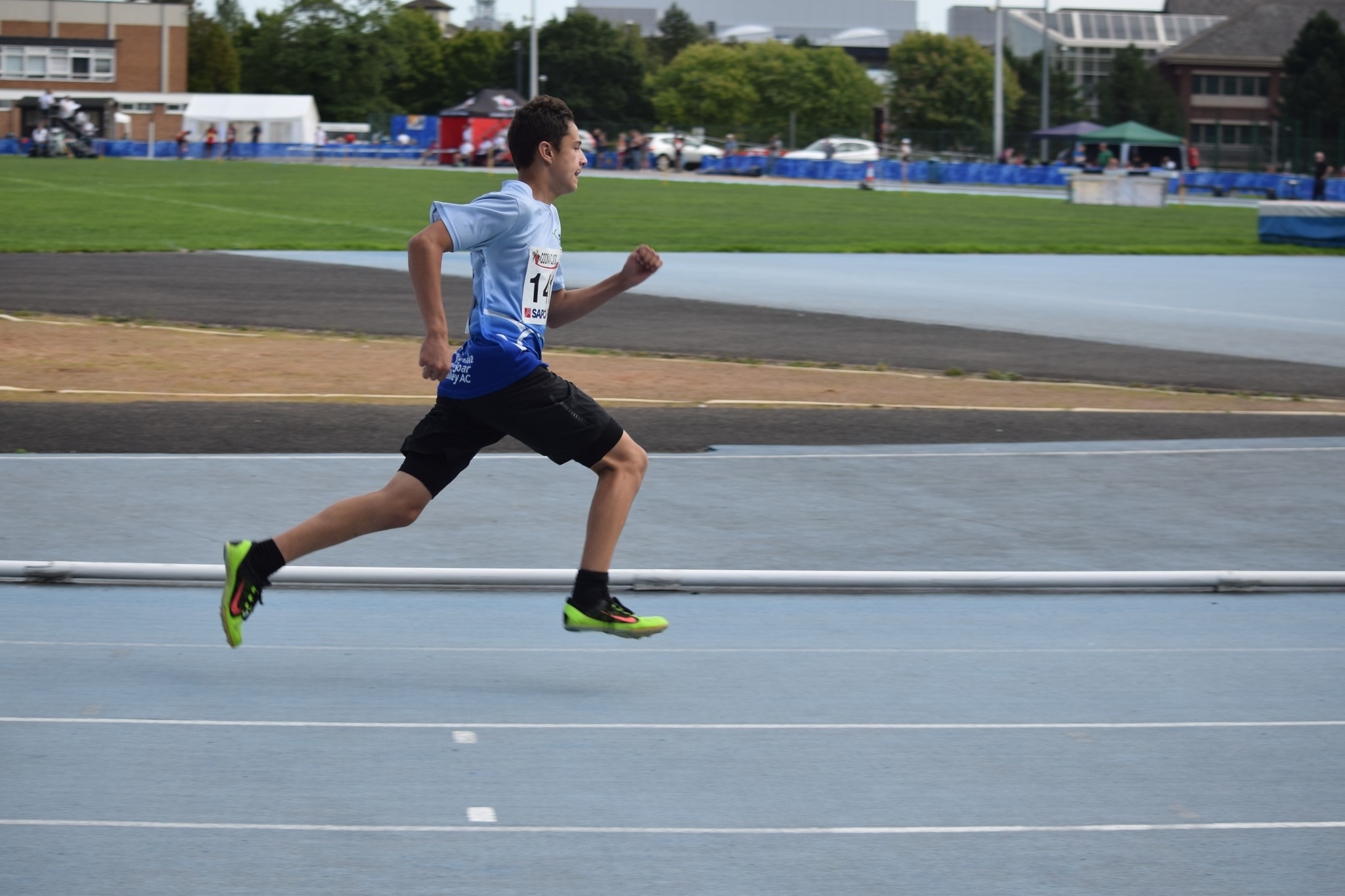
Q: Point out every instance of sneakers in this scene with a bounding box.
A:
[563,595,668,639]
[220,539,263,647]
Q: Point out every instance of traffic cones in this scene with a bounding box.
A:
[860,161,876,191]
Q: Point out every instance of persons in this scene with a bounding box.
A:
[1023,142,1200,176]
[174,121,262,158]
[724,132,737,157]
[767,133,781,158]
[821,140,836,160]
[998,142,1017,165]
[314,126,514,167]
[899,138,913,161]
[221,98,669,649]
[1313,150,1333,201]
[590,126,689,176]
[26,87,100,159]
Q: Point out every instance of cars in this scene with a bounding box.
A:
[782,137,880,164]
[623,132,725,172]
[577,129,598,154]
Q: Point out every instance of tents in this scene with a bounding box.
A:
[1078,120,1182,169]
[1028,120,1107,163]
[438,88,526,119]
